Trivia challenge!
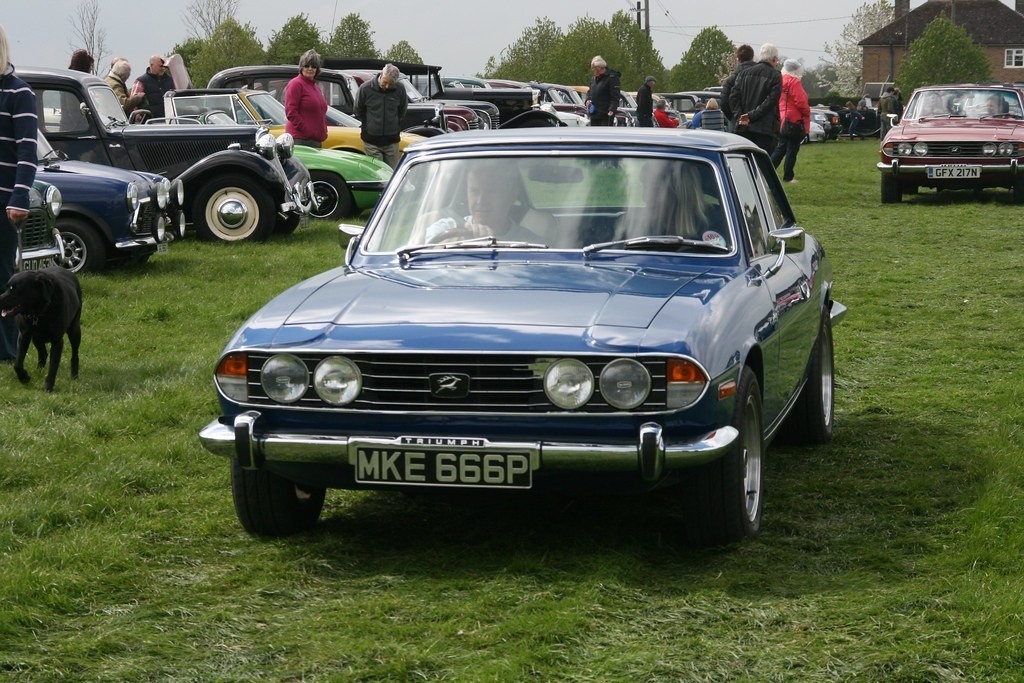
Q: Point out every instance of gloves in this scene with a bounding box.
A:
[862,117,865,120]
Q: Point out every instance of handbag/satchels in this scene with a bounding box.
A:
[780,117,805,138]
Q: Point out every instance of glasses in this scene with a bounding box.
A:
[302,64,318,69]
[91,64,94,68]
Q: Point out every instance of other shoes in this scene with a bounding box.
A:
[789,179,798,183]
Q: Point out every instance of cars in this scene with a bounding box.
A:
[629,86,841,142]
[164,89,429,169]
[877,84,1024,204]
[126,109,396,221]
[14,130,186,274]
[198,125,848,541]
[440,78,686,134]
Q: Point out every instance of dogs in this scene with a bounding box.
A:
[0,267,82,392]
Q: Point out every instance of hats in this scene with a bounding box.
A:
[646,76,656,82]
[783,59,802,71]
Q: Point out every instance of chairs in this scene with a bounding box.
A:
[416,168,560,250]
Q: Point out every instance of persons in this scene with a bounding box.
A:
[354,64,408,169]
[422,156,552,245]
[60,48,176,133]
[637,44,810,183]
[285,49,329,149]
[613,156,726,246]
[585,56,621,127]
[0,23,38,364]
[847,87,1015,142]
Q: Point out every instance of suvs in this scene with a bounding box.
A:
[207,55,499,138]
[9,66,313,247]
